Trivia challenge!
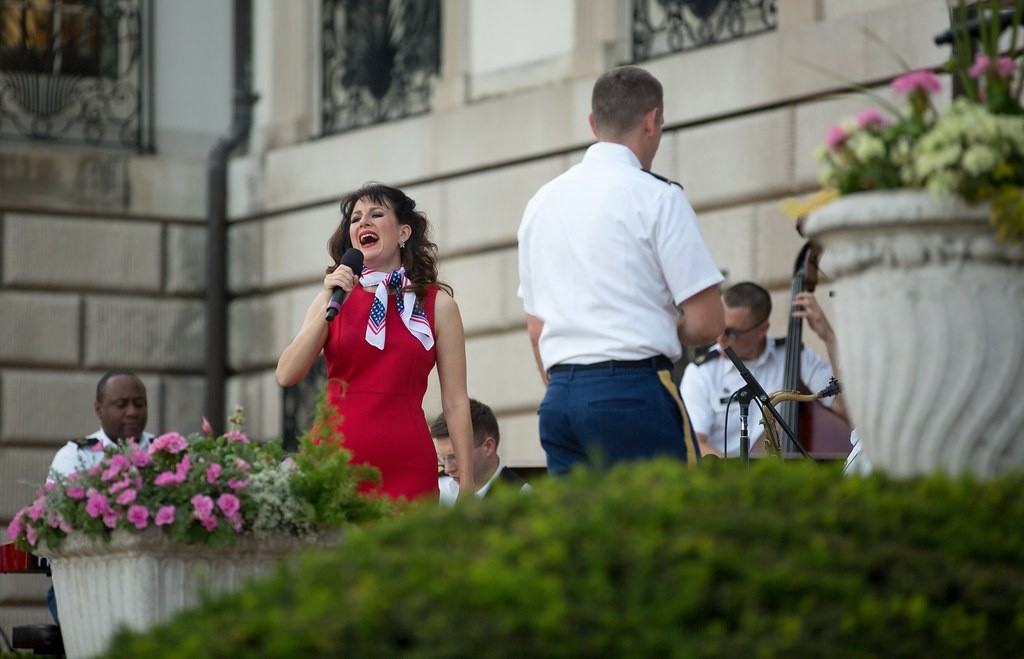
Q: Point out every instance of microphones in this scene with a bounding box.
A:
[324,248,365,322]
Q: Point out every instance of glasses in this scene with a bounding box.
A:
[437,441,483,463]
[725,322,761,339]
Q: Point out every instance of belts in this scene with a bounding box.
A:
[548,356,673,376]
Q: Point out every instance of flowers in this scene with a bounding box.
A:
[787,0,1024,241]
[8,381,388,548]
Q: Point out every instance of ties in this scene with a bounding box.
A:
[359,265,434,352]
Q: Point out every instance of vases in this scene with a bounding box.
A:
[33,528,319,659]
[801,196,1024,486]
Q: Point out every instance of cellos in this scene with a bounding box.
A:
[746,240,854,458]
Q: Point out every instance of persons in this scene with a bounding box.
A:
[274,182,531,518]
[32,370,162,629]
[679,281,853,458]
[517,66,724,478]
